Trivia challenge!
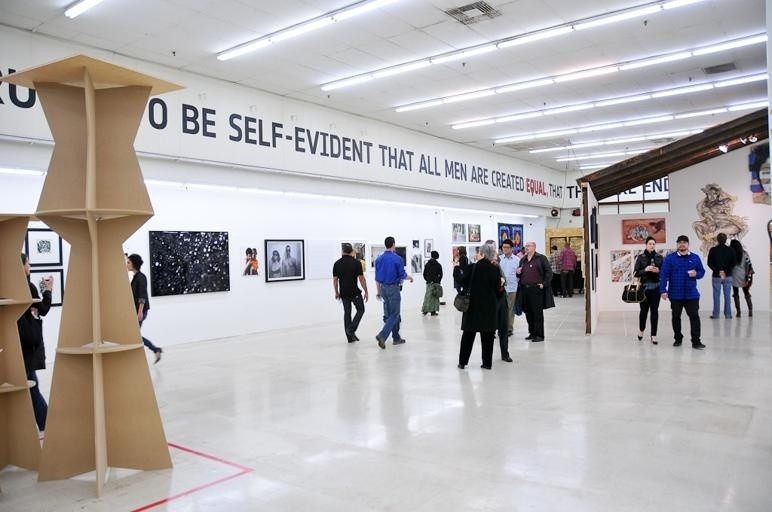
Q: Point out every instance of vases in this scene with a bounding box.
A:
[27,269,63,306]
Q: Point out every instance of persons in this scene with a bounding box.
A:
[242,248,258,275]
[269,250,283,278]
[453,239,577,369]
[127,254,162,364]
[622,237,664,344]
[706,233,737,319]
[281,245,296,276]
[421,251,443,316]
[17,254,53,431]
[374,237,413,350]
[730,240,754,317]
[383,279,403,323]
[659,235,705,348]
[333,243,368,343]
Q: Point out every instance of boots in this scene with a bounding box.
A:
[732,294,742,318]
[744,294,753,317]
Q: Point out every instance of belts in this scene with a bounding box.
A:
[520,283,540,288]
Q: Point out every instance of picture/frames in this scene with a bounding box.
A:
[25,229,63,266]
[367,244,410,272]
[424,260,429,264]
[424,239,434,258]
[264,239,305,282]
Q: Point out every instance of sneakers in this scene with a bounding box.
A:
[525,334,545,342]
[421,312,439,317]
[710,313,733,319]
[673,338,683,347]
[344,328,360,344]
[481,365,492,370]
[502,355,513,362]
[376,333,387,350]
[392,338,406,345]
[457,363,465,370]
[691,338,707,350]
[553,292,574,299]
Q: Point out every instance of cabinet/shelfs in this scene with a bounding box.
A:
[0,214,42,472]
[0,54,187,497]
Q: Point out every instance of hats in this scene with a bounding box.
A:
[676,235,690,244]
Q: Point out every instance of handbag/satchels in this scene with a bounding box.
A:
[431,283,443,298]
[622,270,647,304]
[453,263,477,314]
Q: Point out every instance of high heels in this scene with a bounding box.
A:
[650,334,658,346]
[637,331,644,341]
[154,347,165,364]
